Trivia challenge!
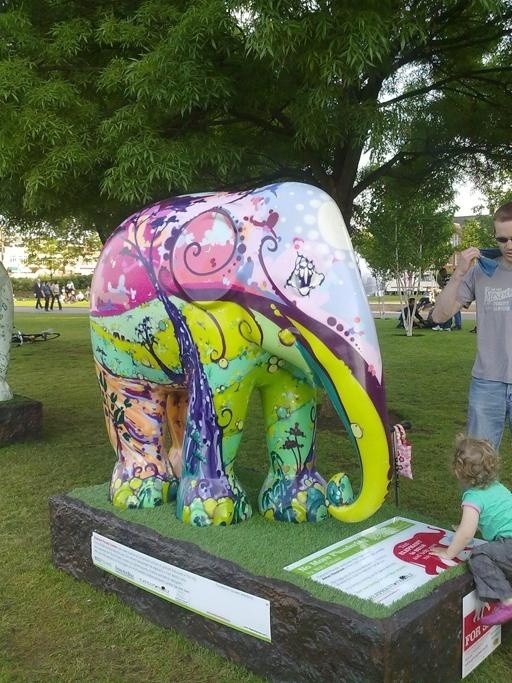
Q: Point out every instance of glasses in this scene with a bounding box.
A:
[494,235,512,244]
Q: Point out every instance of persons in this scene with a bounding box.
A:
[430,201,512,456]
[396,278,462,331]
[32,278,91,312]
[427,432,512,626]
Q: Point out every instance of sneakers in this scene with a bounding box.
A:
[431,323,451,331]
[478,598,512,625]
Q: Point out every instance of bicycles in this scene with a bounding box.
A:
[11,325,60,347]
